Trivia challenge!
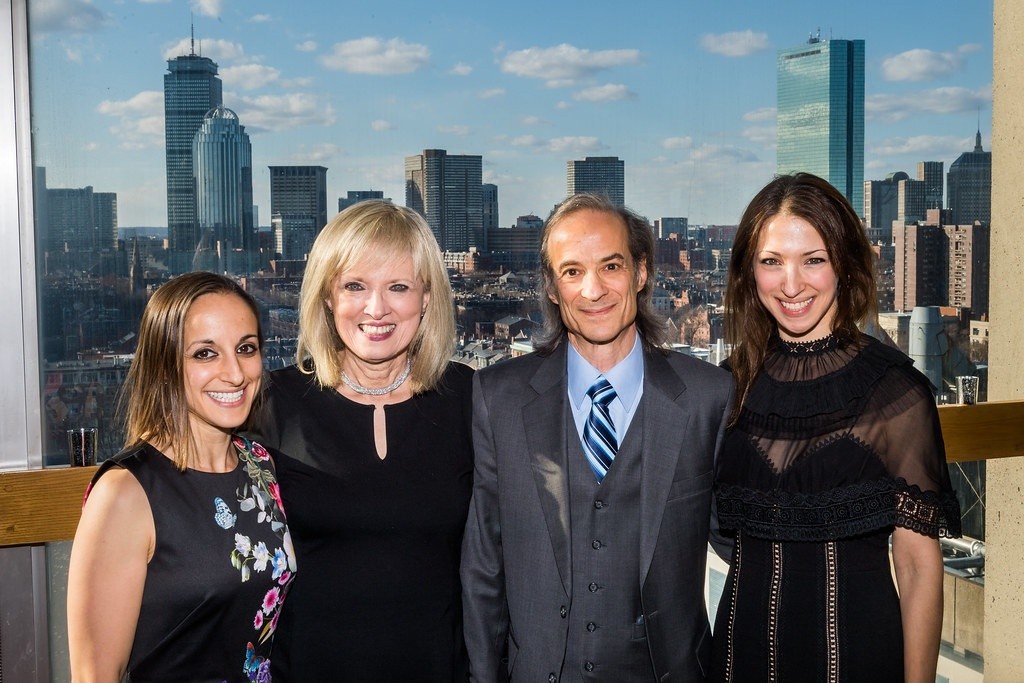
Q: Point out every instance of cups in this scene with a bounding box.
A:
[955,375,979,405]
[67,428,98,467]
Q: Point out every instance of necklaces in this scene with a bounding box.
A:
[341,358,411,395]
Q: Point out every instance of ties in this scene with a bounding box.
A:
[580,376,619,485]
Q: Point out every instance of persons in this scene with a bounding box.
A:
[459,192,735,683]
[237,198,476,683]
[67,273,298,683]
[708,172,964,683]
